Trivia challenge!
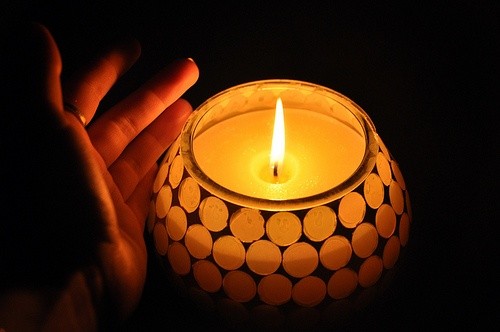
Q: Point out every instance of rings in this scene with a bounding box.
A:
[63,101,87,129]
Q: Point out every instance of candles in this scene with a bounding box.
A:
[146,77,415,320]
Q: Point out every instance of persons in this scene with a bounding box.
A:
[0,20,200,331]
